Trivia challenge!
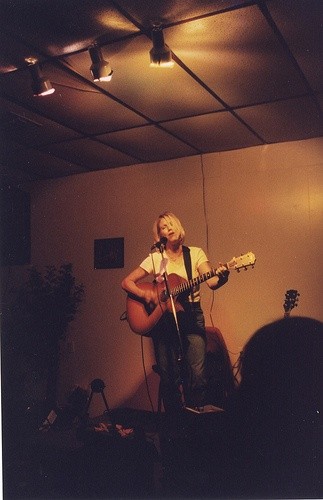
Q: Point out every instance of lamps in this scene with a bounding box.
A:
[88,47,113,82]
[149,29,174,68]
[29,63,55,96]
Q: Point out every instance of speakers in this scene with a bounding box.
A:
[0,187,31,266]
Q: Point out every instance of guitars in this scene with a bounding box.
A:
[125,249,257,337]
[282,288,301,318]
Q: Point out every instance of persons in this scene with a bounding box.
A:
[122,212,229,413]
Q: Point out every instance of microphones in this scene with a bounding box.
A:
[151,237,167,250]
